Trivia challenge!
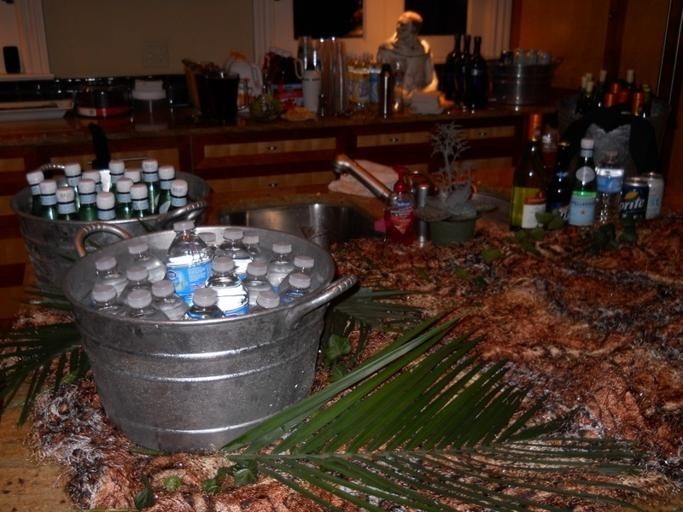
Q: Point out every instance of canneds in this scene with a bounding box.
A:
[643,171,665,222]
[619,177,650,221]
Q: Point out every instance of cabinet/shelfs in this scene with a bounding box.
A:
[1,124,188,268]
[187,116,350,242]
[351,113,514,227]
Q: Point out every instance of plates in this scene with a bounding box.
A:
[238,108,288,121]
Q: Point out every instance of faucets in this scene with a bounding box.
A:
[333,152,430,240]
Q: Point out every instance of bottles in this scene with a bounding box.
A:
[468,35,488,109]
[302,69,321,113]
[296,34,316,75]
[378,63,395,119]
[578,69,652,118]
[445,34,461,100]
[567,137,598,227]
[455,35,472,103]
[90,222,315,321]
[546,140,574,229]
[594,148,626,227]
[28,161,189,221]
[316,38,349,115]
[509,109,548,232]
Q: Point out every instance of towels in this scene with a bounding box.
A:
[327,160,399,198]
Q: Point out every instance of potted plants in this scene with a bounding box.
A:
[420,122,482,244]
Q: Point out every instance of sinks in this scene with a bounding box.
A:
[220,202,371,268]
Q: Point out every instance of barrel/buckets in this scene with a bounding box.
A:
[495,61,548,107]
[10,164,206,300]
[64,222,361,453]
